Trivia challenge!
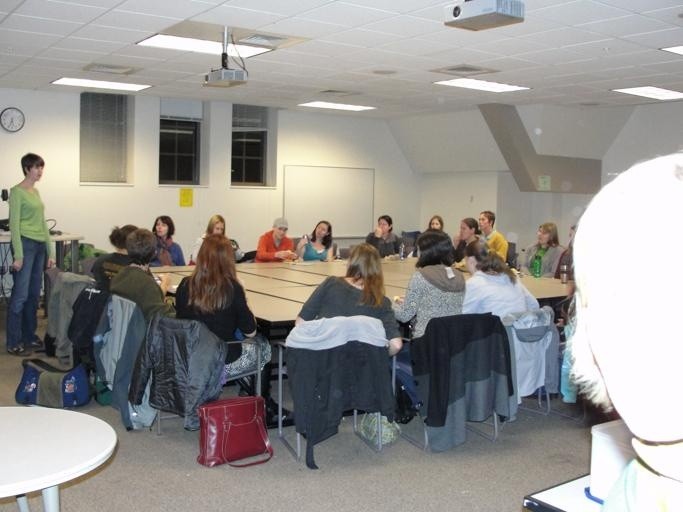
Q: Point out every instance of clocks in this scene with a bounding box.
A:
[1,107,26,133]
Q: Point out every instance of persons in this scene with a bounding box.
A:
[5,152,53,356]
[388,228,466,412]
[365,215,405,258]
[293,220,337,263]
[429,215,452,247]
[90,214,297,427]
[451,212,509,273]
[296,244,403,357]
[518,222,576,343]
[462,239,540,322]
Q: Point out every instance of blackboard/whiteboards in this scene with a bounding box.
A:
[282,164,376,243]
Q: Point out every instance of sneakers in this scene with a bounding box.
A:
[22,338,44,349]
[7,344,32,357]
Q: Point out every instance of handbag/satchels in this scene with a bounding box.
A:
[361,411,402,452]
[15,358,93,409]
[194,396,274,470]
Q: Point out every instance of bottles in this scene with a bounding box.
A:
[304,235,310,245]
[534,254,542,278]
[153,274,163,287]
[560,264,568,284]
[519,249,529,278]
[398,242,406,261]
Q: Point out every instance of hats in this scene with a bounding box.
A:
[272,217,290,231]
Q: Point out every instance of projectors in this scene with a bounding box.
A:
[201,68,248,88]
[443,0,525,32]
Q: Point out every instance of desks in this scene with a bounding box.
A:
[0,228,85,318]
[0,404,119,512]
